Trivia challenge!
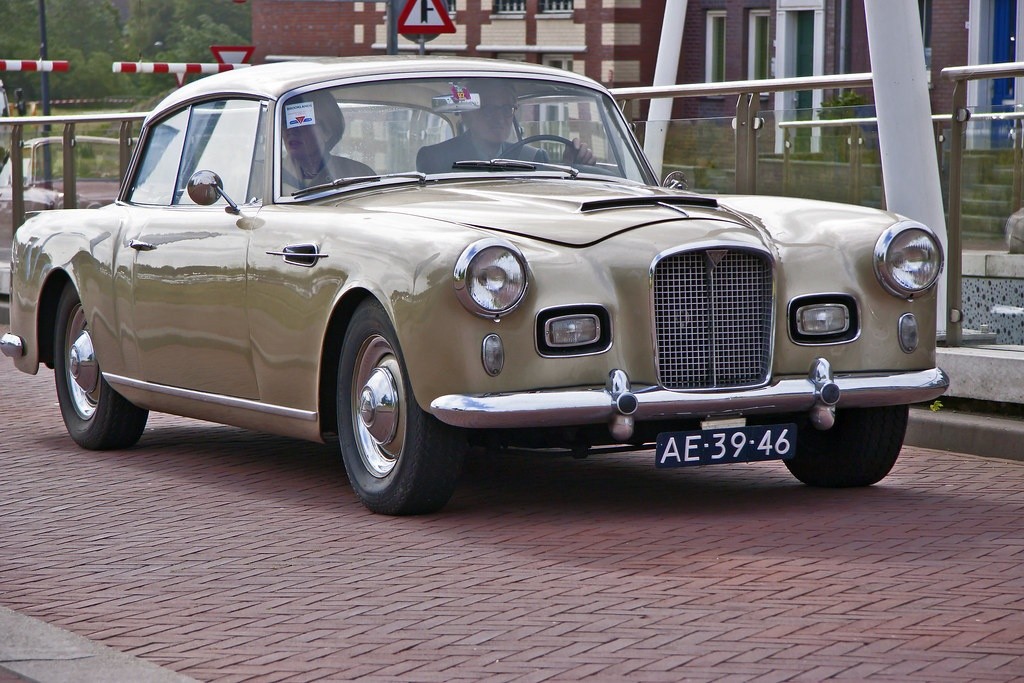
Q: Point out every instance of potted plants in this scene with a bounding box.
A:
[814,87,867,164]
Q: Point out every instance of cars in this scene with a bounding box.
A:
[1,54,954,511]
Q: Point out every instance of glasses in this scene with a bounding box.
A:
[473,102,517,119]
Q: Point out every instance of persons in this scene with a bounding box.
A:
[238,92,379,204]
[0,147,6,175]
[438,79,598,174]
